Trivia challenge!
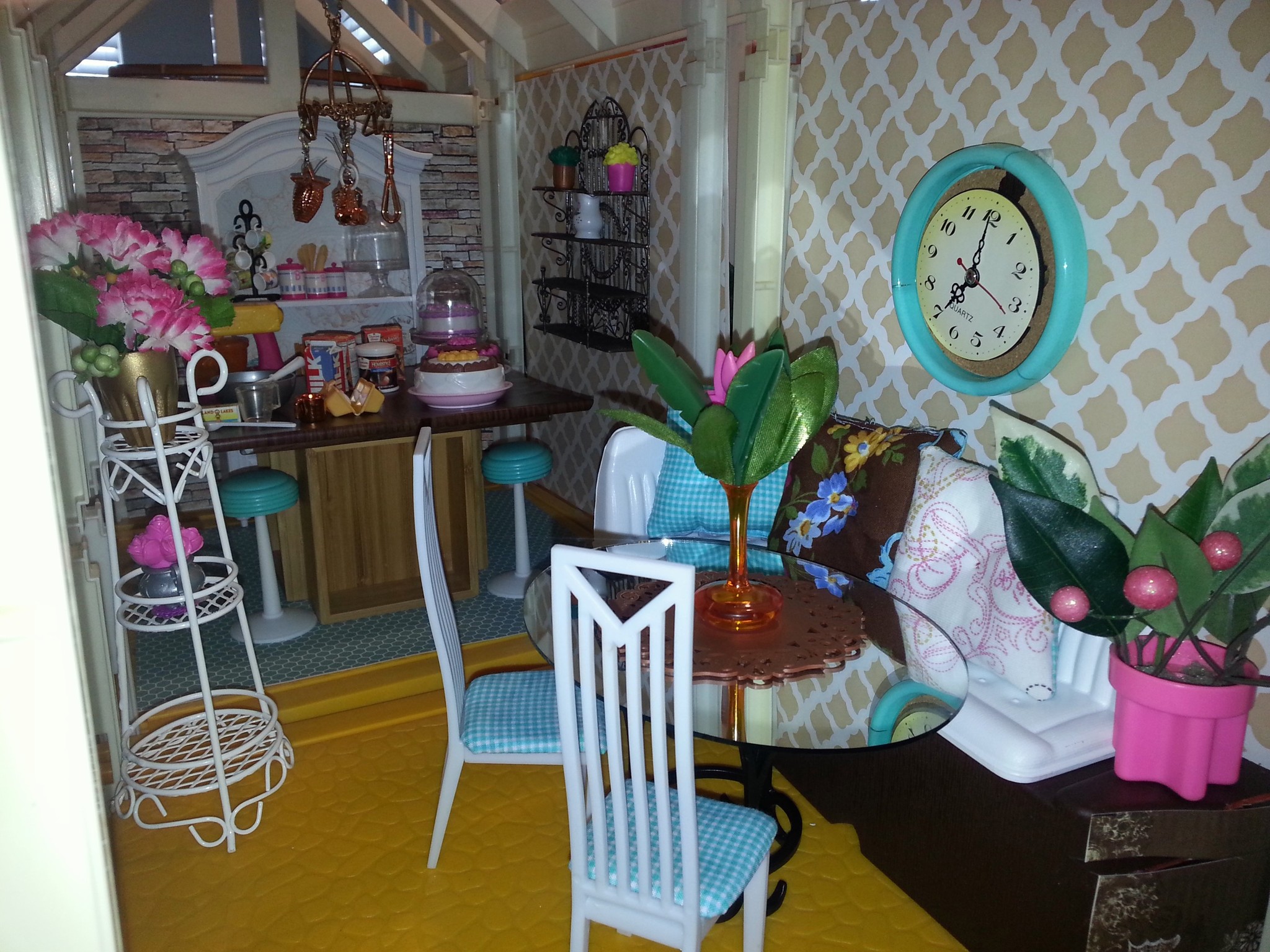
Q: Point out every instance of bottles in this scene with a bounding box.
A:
[571,193,604,239]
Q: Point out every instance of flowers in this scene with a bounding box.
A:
[27,210,236,569]
[597,322,840,488]
[602,141,639,167]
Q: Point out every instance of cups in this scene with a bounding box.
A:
[275,258,347,301]
[234,382,282,422]
[221,223,278,291]
[294,393,326,424]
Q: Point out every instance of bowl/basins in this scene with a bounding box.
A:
[210,371,297,408]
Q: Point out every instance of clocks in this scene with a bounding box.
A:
[866,678,964,745]
[891,141,1089,397]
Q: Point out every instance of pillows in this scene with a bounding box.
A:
[885,446,1062,705]
[646,401,789,536]
[768,415,968,585]
[664,541,907,666]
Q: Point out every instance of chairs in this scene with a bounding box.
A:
[412,425,606,872]
[549,541,777,952]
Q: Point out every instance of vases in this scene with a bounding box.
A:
[91,349,180,448]
[139,557,206,616]
[694,479,785,631]
[726,684,747,742]
[572,193,603,239]
[607,162,635,191]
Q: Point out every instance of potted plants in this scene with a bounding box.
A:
[547,145,583,189]
[984,401,1270,804]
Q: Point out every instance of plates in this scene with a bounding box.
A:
[407,381,513,409]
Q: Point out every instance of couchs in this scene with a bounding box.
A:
[598,424,1115,949]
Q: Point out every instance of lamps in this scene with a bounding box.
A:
[290,0,404,226]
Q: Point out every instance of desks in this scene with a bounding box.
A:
[1015,756,1270,952]
[524,539,969,925]
[186,364,593,625]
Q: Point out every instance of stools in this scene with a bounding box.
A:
[481,432,555,600]
[217,468,318,644]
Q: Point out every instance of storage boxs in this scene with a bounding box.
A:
[304,324,404,394]
[190,404,242,423]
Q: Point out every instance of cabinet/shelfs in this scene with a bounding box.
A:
[531,97,652,354]
[47,341,301,858]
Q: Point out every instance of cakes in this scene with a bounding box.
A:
[418,302,482,334]
[414,336,504,394]
